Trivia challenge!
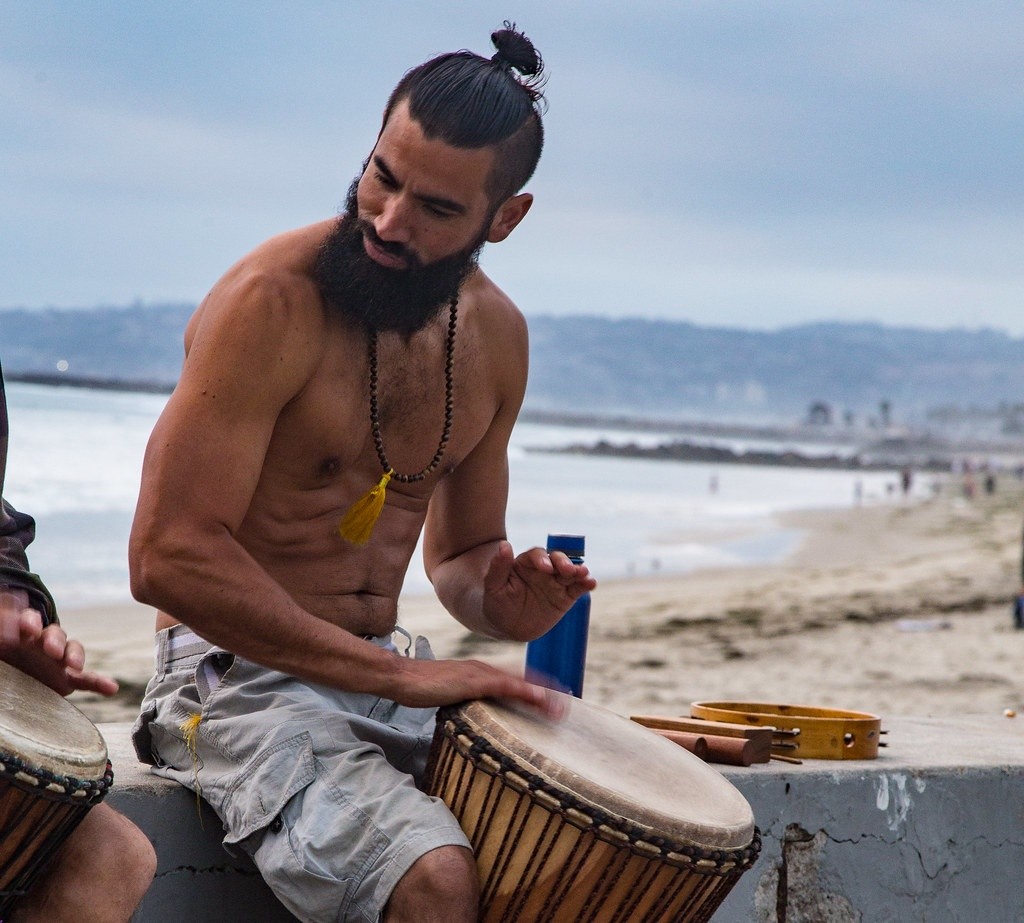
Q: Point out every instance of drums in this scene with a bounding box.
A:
[425,680,757,923]
[0,657,114,908]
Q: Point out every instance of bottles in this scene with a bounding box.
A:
[522,532,592,698]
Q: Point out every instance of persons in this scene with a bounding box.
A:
[131,25,598,923]
[0,360,157,923]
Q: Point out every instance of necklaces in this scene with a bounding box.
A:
[337,276,458,550]
